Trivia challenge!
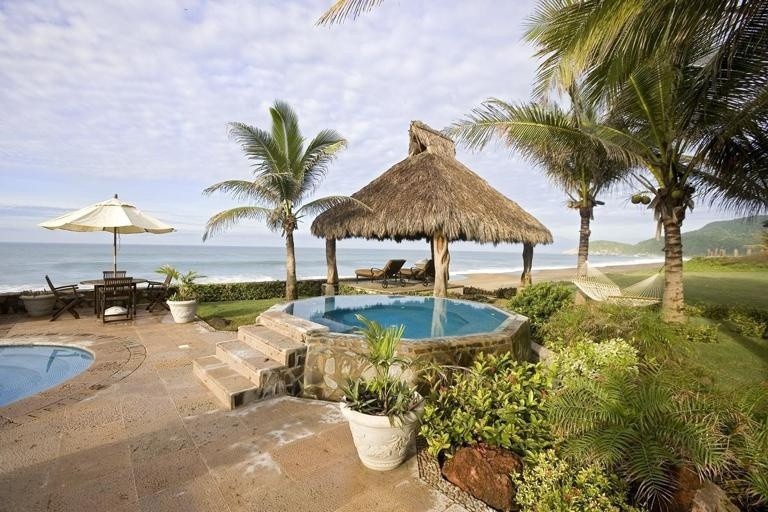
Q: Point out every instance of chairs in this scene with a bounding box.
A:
[45,271,171,324]
[354,259,434,291]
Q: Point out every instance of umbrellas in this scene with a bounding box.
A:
[37,192,178,295]
[311,146,552,298]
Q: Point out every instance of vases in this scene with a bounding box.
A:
[17,288,58,317]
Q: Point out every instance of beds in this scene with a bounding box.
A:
[569,261,676,307]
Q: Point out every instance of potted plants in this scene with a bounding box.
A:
[339,313,426,471]
[155,264,208,323]
